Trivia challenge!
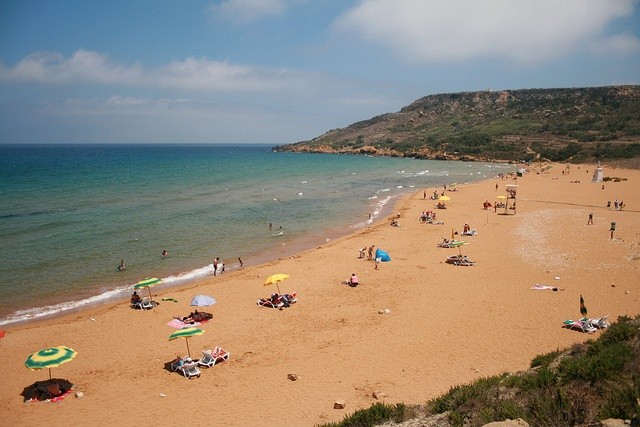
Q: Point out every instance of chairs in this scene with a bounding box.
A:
[460,229,477,236]
[569,318,596,334]
[271,294,297,303]
[199,350,229,368]
[454,262,475,266]
[257,298,284,309]
[130,297,153,311]
[591,315,610,329]
[170,361,201,379]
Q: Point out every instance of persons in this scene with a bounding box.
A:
[437,238,455,248]
[494,201,497,211]
[221,263,225,274]
[173,356,192,368]
[131,291,142,303]
[173,309,209,324]
[615,199,618,208]
[373,259,378,269]
[419,210,436,223]
[495,183,499,189]
[257,292,290,312]
[47,380,70,398]
[483,200,491,209]
[213,257,219,275]
[391,219,400,227]
[587,211,593,225]
[619,201,623,205]
[238,257,243,268]
[207,346,225,358]
[350,273,358,287]
[119,260,125,270]
[161,249,168,258]
[607,201,611,207]
[444,184,458,191]
[617,204,621,209]
[424,190,439,200]
[464,222,470,233]
[359,246,367,258]
[441,191,444,195]
[368,245,374,260]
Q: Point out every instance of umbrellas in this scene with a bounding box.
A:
[496,195,507,205]
[168,326,205,358]
[438,196,450,205]
[191,295,216,307]
[451,227,455,241]
[134,277,162,302]
[23,346,79,381]
[449,240,466,257]
[263,273,289,294]
[579,294,588,320]
[375,249,390,261]
